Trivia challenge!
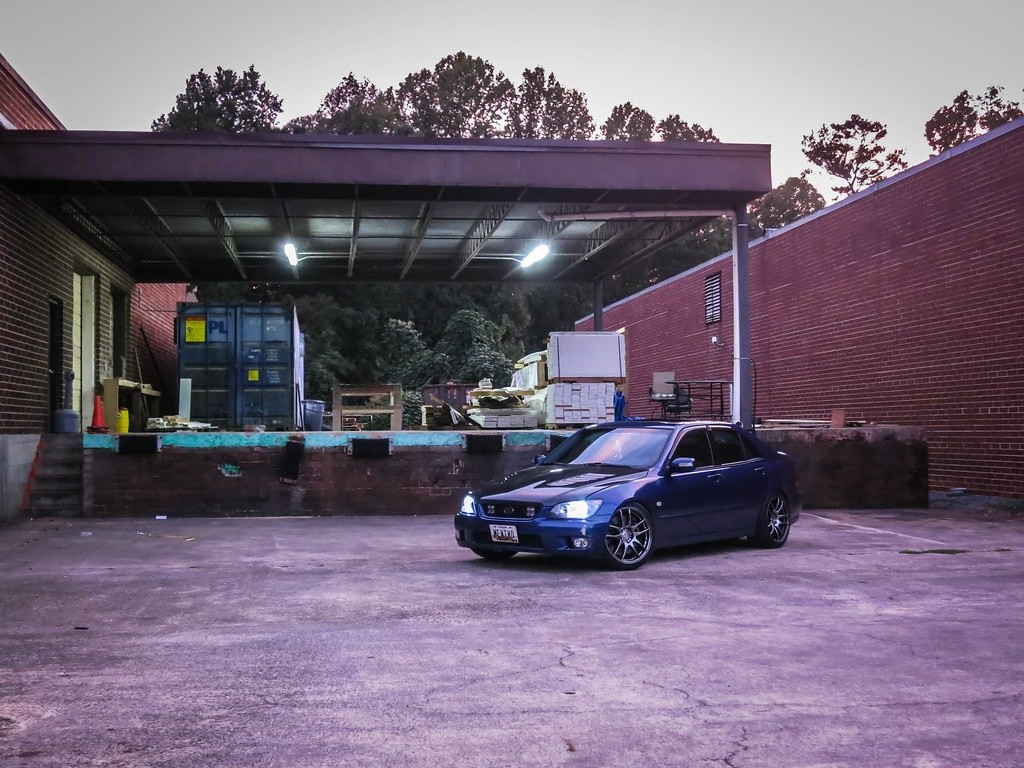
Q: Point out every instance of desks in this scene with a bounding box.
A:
[664,381,733,419]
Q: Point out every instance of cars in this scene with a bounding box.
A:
[454,419,801,570]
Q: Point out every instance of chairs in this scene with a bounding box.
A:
[664,386,693,420]
[648,372,676,419]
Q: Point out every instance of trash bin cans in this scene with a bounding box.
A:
[301,400,326,430]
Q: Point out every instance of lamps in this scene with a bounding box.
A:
[520,245,550,268]
[283,242,298,267]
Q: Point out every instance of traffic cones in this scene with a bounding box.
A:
[87,395,110,434]
